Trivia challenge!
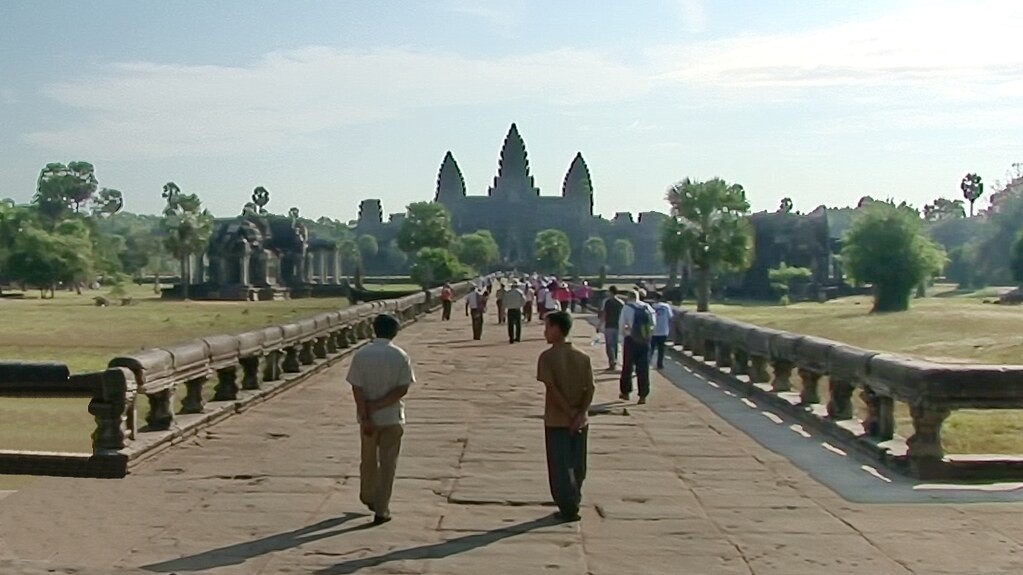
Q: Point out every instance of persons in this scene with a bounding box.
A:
[578,281,592,313]
[537,313,595,521]
[504,281,526,344]
[346,314,416,523]
[466,270,576,324]
[282,208,307,283]
[466,286,488,340]
[596,286,651,370]
[651,291,673,368]
[442,280,456,321]
[619,291,656,404]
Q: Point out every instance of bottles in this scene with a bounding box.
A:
[591,337,599,345]
[624,324,630,336]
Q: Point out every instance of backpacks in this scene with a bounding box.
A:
[625,303,652,344]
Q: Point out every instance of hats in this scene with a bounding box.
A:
[633,287,647,297]
[558,281,568,289]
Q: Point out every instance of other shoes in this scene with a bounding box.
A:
[473,337,480,340]
[606,362,616,370]
[503,319,505,322]
[553,511,581,523]
[499,322,501,324]
[373,514,392,525]
[657,364,663,369]
[619,392,629,400]
[510,339,521,344]
[638,399,646,404]
